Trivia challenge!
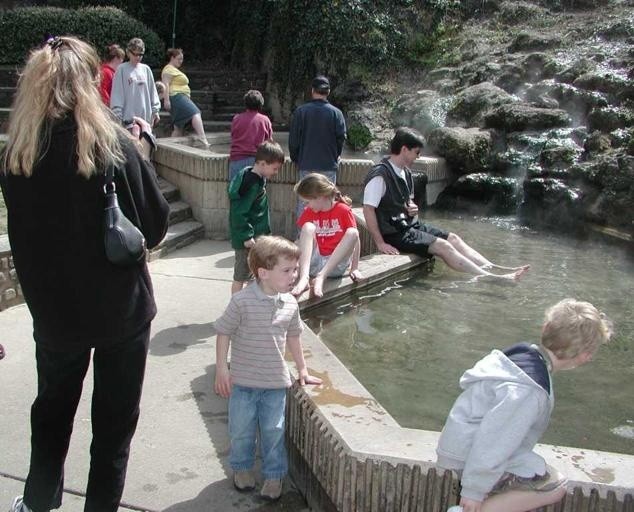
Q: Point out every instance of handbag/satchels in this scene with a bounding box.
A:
[103,189,147,269]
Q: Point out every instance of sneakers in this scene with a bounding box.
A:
[234,472,256,492]
[260,479,283,502]
[13,494,32,512]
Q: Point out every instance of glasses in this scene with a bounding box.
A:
[129,49,145,56]
[415,150,420,156]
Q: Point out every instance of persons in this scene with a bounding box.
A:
[155,80,166,101]
[226,140,284,296]
[159,47,210,147]
[436,297,614,512]
[96,43,125,106]
[291,172,366,297]
[362,125,531,283]
[0,36,171,511]
[107,37,161,133]
[227,89,273,185]
[288,75,348,240]
[210,234,324,499]
[130,116,159,163]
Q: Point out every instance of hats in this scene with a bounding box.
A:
[312,77,329,88]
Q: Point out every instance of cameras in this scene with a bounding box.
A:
[122,120,137,135]
